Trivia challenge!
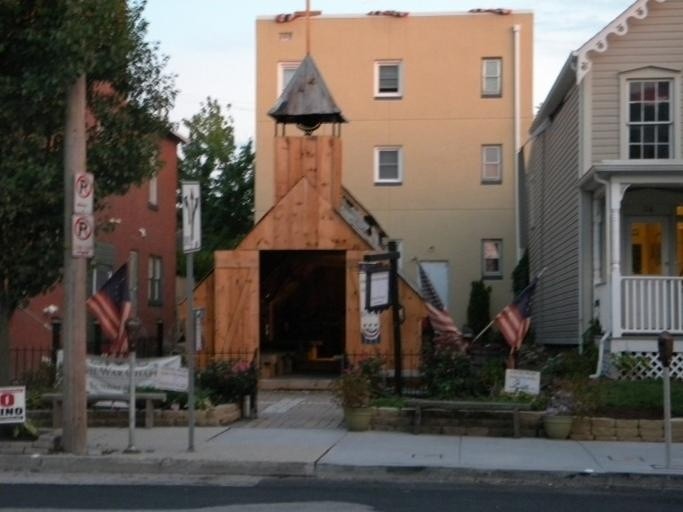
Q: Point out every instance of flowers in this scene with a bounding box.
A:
[537,391,586,421]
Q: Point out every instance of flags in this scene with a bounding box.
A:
[86,261,134,357]
[495,276,535,353]
[418,265,463,351]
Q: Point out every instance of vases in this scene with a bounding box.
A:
[540,414,575,440]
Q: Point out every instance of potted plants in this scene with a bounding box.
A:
[322,372,376,432]
